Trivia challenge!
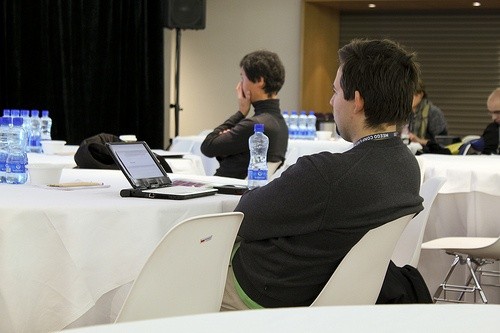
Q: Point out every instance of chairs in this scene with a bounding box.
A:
[113,211,244,325]
[310,176,448,308]
[420,237,500,304]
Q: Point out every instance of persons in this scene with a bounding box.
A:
[220,39,424,312]
[481,87,500,156]
[401,81,448,155]
[201,51,289,180]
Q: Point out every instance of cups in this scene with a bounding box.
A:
[317,131,331,139]
[120,134,137,142]
[25,138,66,187]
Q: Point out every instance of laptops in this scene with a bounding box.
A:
[105,141,215,200]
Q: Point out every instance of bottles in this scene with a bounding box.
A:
[299,111,307,138]
[0,109,51,185]
[289,111,299,138]
[282,111,288,127]
[307,111,317,139]
[247,124,269,189]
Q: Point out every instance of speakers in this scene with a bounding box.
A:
[162,0,207,30]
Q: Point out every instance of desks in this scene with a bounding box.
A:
[417,154,500,304]
[0,136,356,333]
